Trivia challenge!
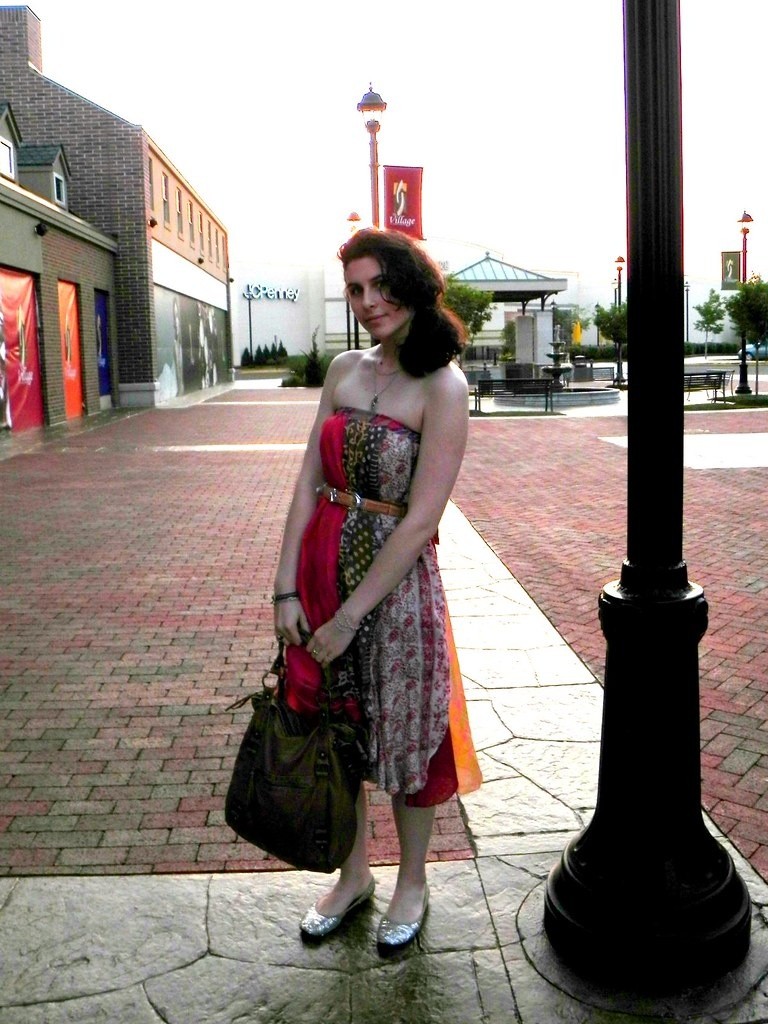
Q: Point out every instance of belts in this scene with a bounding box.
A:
[324,484,407,518]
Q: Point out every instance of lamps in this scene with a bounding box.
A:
[198,257,204,264]
[148,219,158,227]
[33,222,49,236]
[229,278,234,282]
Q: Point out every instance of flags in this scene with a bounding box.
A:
[573,323,581,345]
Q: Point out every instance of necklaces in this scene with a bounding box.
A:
[369,348,409,416]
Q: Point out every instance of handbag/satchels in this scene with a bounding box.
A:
[224,631,362,873]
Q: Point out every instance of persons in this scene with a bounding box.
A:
[269,227,485,948]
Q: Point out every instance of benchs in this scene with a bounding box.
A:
[684,369,735,404]
[592,367,615,384]
[474,378,555,414]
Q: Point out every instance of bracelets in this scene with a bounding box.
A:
[334,607,360,635]
[272,592,300,603]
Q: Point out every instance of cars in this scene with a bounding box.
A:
[736,336,768,361]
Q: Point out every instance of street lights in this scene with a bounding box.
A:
[736,209,755,395]
[610,255,626,382]
[346,211,362,352]
[684,271,692,342]
[356,81,388,229]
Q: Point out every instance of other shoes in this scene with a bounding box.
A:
[300,875,375,935]
[378,883,429,945]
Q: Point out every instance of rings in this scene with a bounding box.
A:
[314,648,318,656]
[327,656,332,663]
